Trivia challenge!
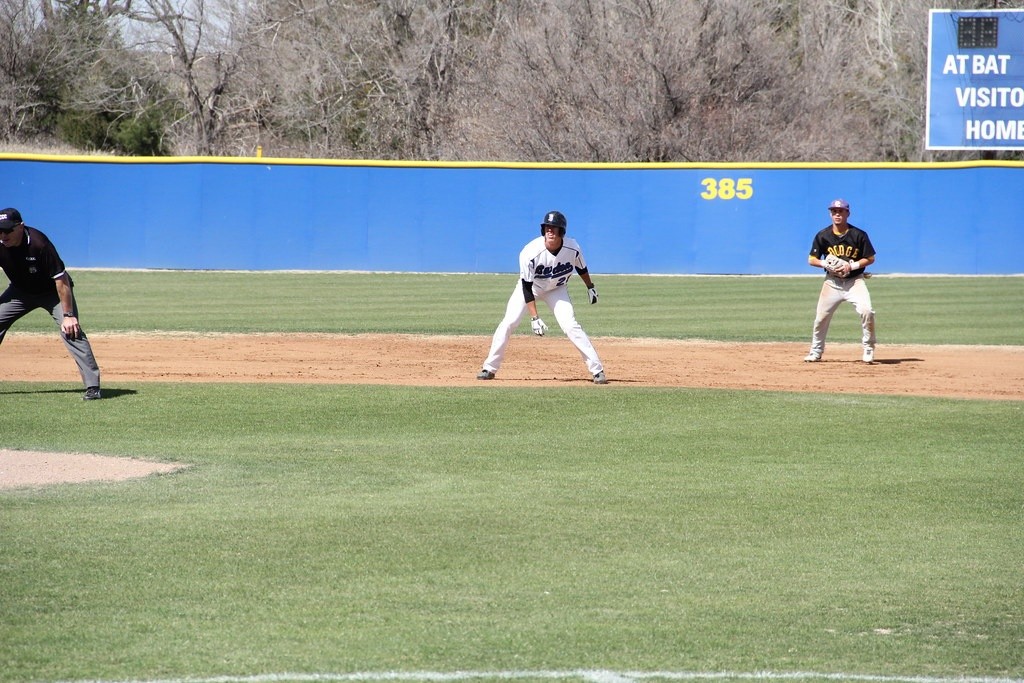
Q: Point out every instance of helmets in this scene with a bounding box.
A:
[539,210,567,237]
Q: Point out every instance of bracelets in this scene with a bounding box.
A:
[587,283,594,289]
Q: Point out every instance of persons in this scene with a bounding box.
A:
[805,198,876,363]
[0,208,101,401]
[476,211,607,384]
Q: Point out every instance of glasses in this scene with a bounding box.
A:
[0,223,20,233]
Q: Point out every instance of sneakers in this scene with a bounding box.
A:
[594,370,608,384]
[83,386,101,399]
[477,368,495,380]
[804,352,821,362]
[863,347,874,362]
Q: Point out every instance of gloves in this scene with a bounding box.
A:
[530,316,548,336]
[587,284,598,303]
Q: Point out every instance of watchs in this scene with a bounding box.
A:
[63,312,73,317]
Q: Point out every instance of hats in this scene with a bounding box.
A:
[0,207,22,229]
[828,199,850,211]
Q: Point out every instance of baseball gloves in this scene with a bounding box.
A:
[824,254,850,278]
